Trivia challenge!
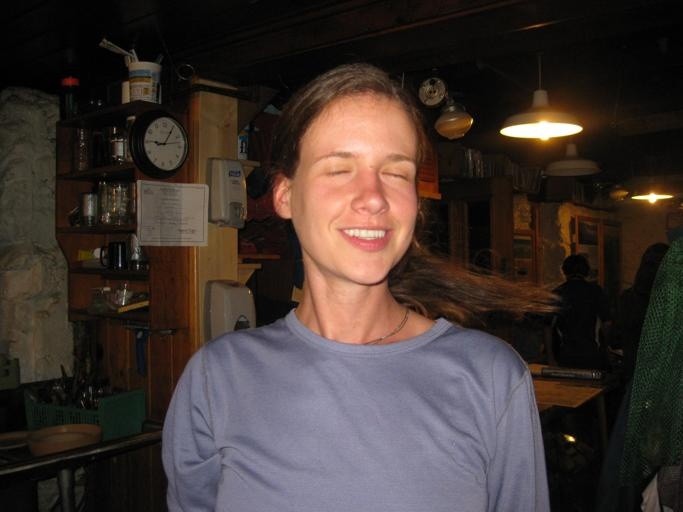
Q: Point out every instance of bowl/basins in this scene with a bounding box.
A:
[26,423,103,459]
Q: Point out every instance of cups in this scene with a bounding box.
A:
[100,241,131,274]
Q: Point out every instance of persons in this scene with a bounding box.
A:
[543,252,615,456]
[157,61,565,512]
[617,240,669,392]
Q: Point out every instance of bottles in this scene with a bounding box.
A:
[79,178,136,227]
[55,75,83,121]
[73,124,133,171]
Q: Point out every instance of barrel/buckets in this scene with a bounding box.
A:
[129,62,161,102]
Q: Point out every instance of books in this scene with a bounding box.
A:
[105,293,150,313]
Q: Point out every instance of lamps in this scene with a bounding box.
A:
[498,43,584,143]
[419,74,475,153]
[545,142,599,181]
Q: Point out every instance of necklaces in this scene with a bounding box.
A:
[364,307,411,346]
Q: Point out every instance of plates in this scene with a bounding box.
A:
[0,430,33,450]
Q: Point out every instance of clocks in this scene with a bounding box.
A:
[129,109,190,180]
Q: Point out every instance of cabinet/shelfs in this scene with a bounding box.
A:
[57,106,151,326]
